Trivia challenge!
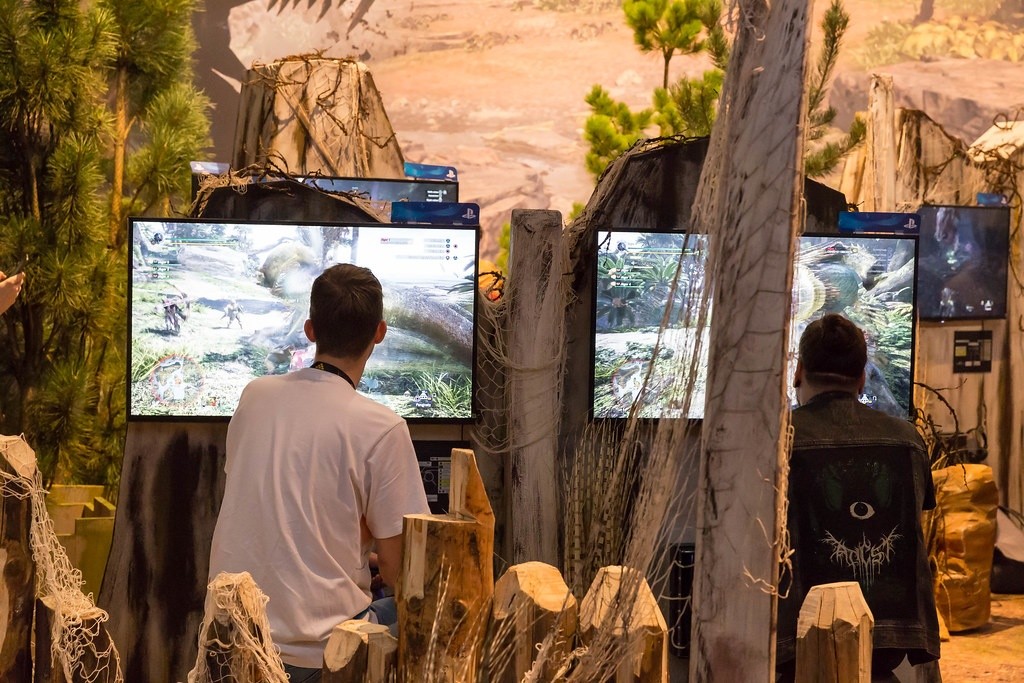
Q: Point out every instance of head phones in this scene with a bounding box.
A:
[965,428,988,462]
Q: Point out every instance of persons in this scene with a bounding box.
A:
[197,263,431,683]
[771,314,941,683]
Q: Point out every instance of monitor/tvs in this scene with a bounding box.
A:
[584,200,1011,423]
[125,174,480,424]
